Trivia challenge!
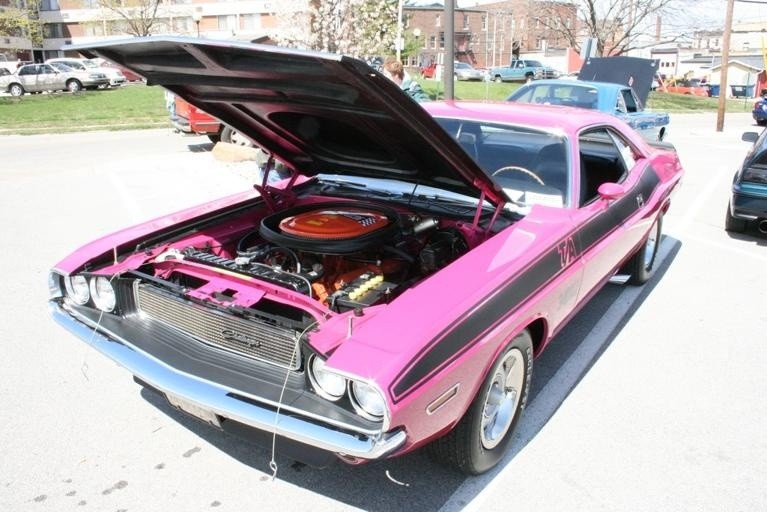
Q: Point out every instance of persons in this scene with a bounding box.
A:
[383,61,431,103]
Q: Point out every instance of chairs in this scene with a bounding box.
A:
[444,121,482,163]
[527,143,587,207]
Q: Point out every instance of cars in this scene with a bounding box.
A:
[0,57,138,98]
[655,68,713,98]
[725,88,767,240]
